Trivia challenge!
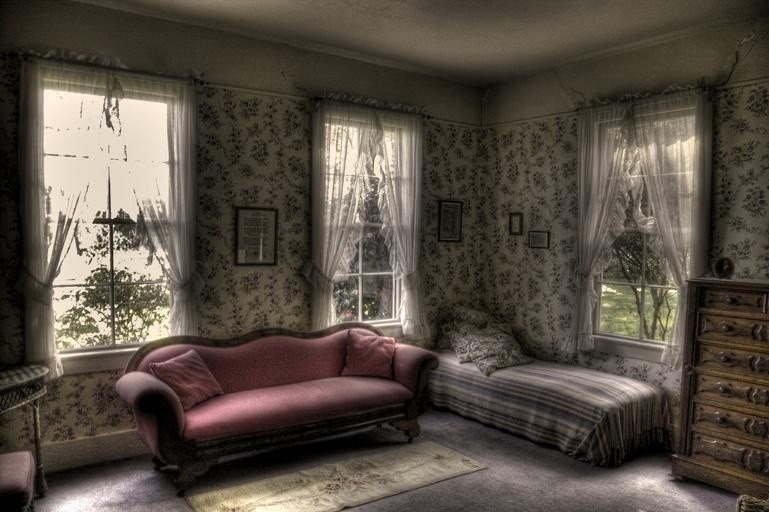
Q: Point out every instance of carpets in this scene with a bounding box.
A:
[181,425,490,511]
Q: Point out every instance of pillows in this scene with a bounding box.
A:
[338,329,397,380]
[148,350,225,411]
[464,324,532,379]
[447,321,482,365]
[445,305,494,330]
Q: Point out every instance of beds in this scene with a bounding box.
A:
[423,339,670,468]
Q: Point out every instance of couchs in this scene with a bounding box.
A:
[113,324,440,499]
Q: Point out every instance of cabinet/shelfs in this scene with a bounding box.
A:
[670,272,768,506]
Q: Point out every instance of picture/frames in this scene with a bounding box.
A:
[436,199,463,244]
[509,211,523,238]
[232,205,278,267]
[527,230,551,250]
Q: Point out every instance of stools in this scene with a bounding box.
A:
[0,442,44,511]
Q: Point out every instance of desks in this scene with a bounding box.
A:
[0,353,58,502]
